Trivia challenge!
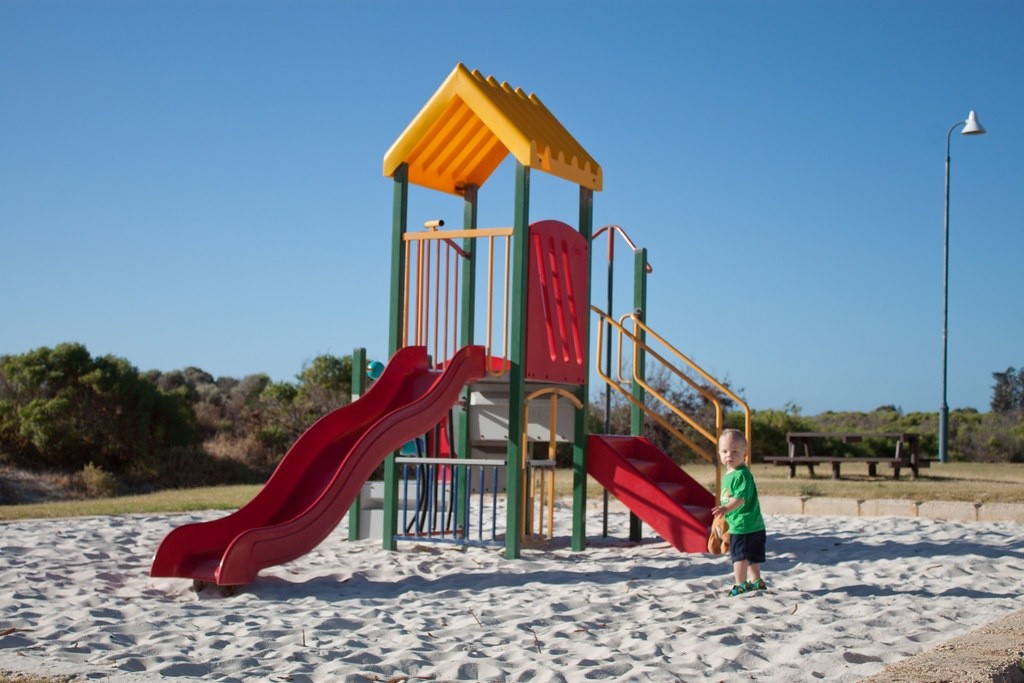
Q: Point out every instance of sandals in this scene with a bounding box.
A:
[745,578,768,589]
[729,583,753,597]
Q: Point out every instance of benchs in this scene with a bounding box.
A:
[763,456,940,462]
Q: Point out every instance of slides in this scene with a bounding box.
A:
[149,343,487,590]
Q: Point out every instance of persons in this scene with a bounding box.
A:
[710,429,767,597]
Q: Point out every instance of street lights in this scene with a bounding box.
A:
[937,109,988,462]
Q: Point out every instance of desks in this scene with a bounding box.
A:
[787,433,919,481]
